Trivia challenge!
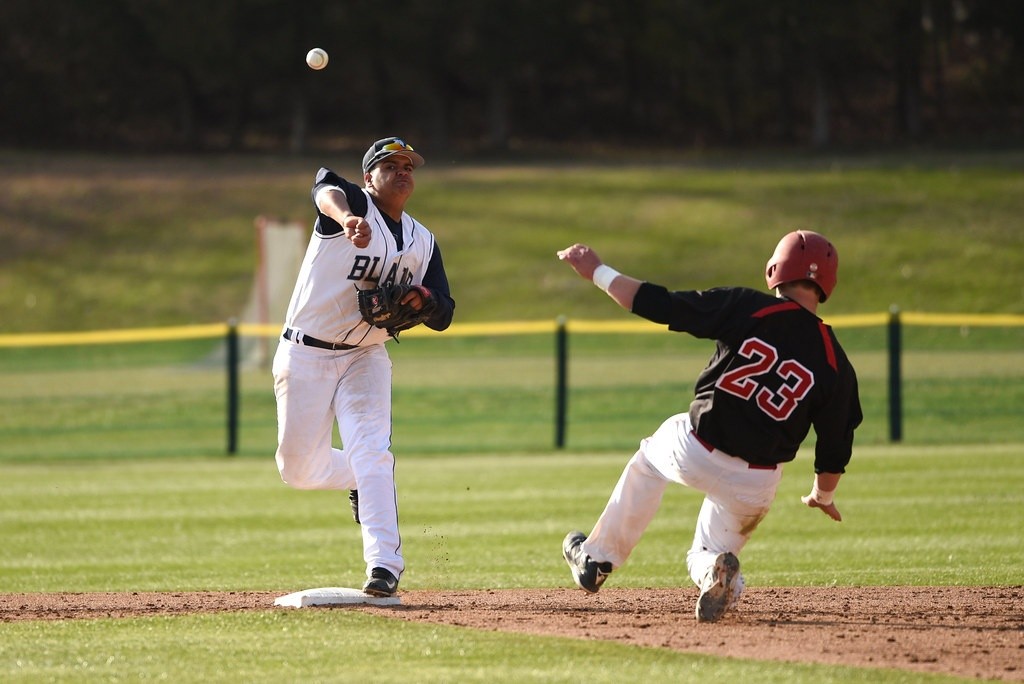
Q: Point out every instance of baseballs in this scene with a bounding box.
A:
[306,48,328,70]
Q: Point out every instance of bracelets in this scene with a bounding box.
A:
[592,263,621,292]
[811,486,837,506]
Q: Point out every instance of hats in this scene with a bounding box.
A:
[361,137,425,175]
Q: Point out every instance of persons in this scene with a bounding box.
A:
[274,137,455,599]
[556,230,864,623]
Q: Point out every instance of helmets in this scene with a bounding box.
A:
[765,230,838,303]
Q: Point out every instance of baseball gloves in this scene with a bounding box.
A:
[357,282,438,335]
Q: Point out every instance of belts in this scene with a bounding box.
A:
[283,329,360,350]
[690,430,778,470]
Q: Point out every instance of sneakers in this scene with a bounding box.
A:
[349,489,360,524]
[363,567,398,597]
[562,530,613,594]
[695,552,741,625]
